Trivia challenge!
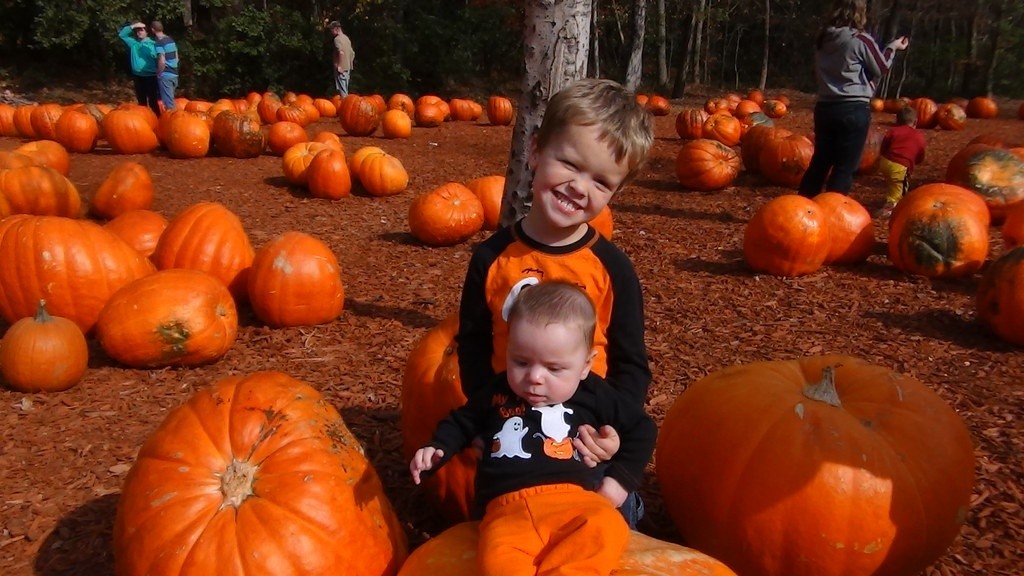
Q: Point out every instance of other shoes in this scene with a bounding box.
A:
[881,201,897,218]
[902,173,909,192]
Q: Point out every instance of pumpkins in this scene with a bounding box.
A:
[657,354,977,576]
[396,518,735,576]
[400,314,479,519]
[109,371,410,576]
[630,91,1024,349]
[0,90,515,392]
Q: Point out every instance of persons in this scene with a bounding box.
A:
[118,20,181,118]
[406,276,664,571]
[326,21,356,99]
[876,105,926,220]
[460,77,654,407]
[798,0,911,198]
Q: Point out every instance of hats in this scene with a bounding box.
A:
[327,20,341,29]
